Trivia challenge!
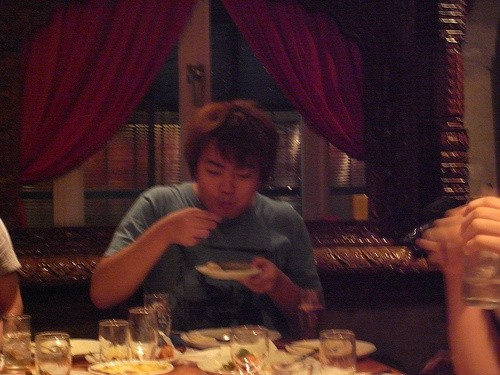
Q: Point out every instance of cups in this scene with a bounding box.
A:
[319,329,359,375]
[127,307,158,360]
[142,292,172,348]
[459,246,500,312]
[229,324,270,375]
[2,313,31,375]
[34,331,71,375]
[98,319,129,361]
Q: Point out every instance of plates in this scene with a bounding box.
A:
[286,338,376,361]
[86,348,183,361]
[30,339,98,358]
[195,345,291,375]
[180,325,282,344]
[194,264,261,281]
[87,361,175,375]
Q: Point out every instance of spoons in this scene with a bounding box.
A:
[272,347,318,373]
[172,330,230,342]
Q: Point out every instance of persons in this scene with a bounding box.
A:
[419,195,500,375]
[91,100,326,340]
[0,217,23,348]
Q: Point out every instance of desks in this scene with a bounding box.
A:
[0,340,406,375]
